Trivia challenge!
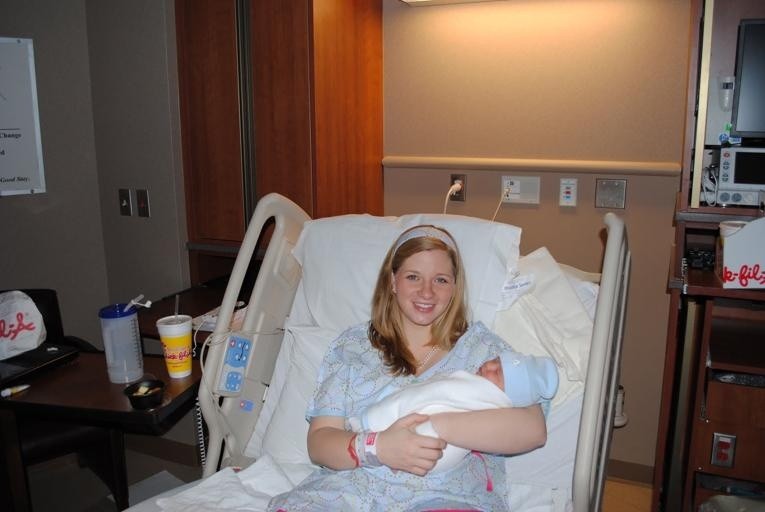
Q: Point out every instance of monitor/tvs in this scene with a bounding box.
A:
[730,17,765,138]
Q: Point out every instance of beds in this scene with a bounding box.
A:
[117,192,631,512]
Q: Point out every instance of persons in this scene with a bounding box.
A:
[345,353,559,472]
[268,225,550,512]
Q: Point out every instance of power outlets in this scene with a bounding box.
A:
[450,173,467,202]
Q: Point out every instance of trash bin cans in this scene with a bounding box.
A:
[701,495,762,512]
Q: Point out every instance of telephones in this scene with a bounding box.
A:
[191,300,248,333]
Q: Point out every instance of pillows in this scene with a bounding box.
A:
[290,210,523,332]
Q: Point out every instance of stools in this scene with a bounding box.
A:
[130,278,246,353]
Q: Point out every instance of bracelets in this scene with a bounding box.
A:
[357,429,382,465]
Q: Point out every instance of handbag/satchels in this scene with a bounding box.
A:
[0,290,48,362]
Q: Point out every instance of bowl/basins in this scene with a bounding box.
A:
[123,381,166,408]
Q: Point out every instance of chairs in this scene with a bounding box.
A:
[2,285,127,509]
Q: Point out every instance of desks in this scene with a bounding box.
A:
[5,354,219,506]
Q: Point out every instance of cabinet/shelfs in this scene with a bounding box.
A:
[648,241,764,512]
[173,0,383,248]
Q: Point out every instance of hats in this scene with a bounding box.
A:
[499,351,560,408]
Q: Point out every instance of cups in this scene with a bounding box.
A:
[156,314,193,379]
[99,303,146,385]
[716,219,748,248]
